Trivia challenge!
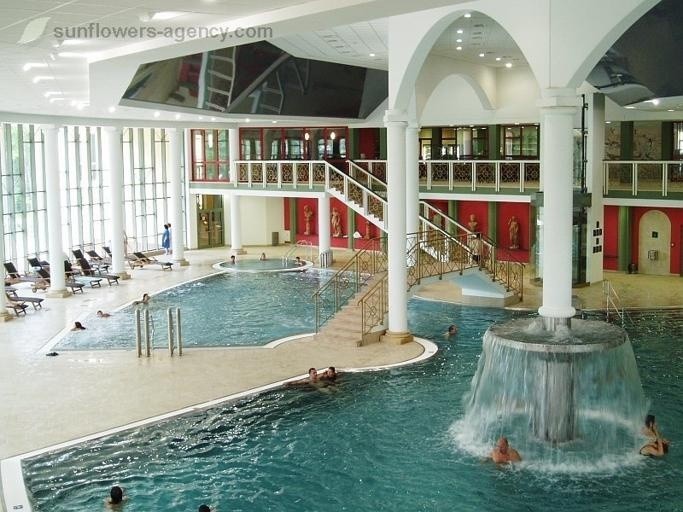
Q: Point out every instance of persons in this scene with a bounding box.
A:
[294,368,338,396]
[294,257,301,266]
[303,204,313,235]
[161,224,169,255]
[506,216,519,250]
[321,366,335,378]
[70,321,86,331]
[467,214,478,233]
[440,325,457,341]
[639,437,668,460]
[642,414,659,437]
[491,438,521,465]
[468,233,482,265]
[167,223,172,254]
[133,293,149,305]
[260,252,265,260]
[330,207,342,237]
[198,504,209,512]
[102,486,129,508]
[96,310,109,319]
[230,255,236,265]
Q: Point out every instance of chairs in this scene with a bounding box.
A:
[3,235,173,318]
